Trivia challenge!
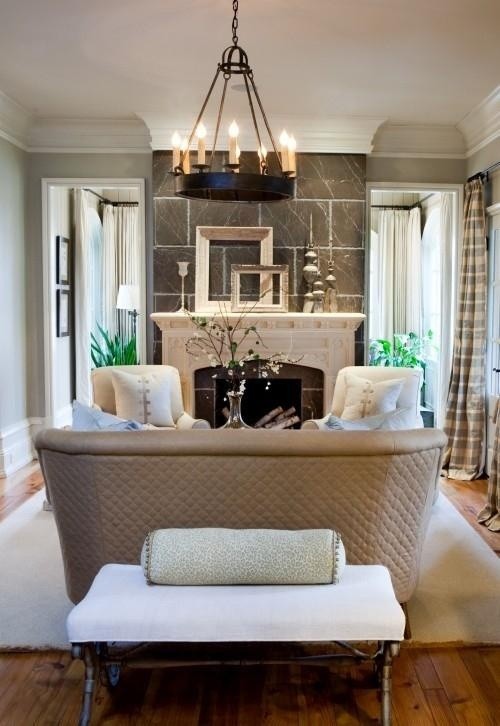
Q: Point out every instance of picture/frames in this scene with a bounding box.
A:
[56,235,71,284]
[57,289,73,337]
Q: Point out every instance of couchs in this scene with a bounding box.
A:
[31,429,449,605]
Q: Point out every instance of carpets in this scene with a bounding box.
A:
[0,489,499,650]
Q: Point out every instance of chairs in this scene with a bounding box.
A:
[91,364,213,430]
[302,365,425,433]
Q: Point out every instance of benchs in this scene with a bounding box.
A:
[65,563,406,725]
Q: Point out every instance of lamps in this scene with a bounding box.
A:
[116,284,139,335]
[167,0,298,204]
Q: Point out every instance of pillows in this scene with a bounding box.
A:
[340,377,405,421]
[140,526,345,587]
[68,398,147,428]
[109,370,178,428]
[329,413,394,431]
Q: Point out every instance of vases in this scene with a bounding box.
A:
[220,392,252,428]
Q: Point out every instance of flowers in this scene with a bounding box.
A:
[176,280,308,394]
